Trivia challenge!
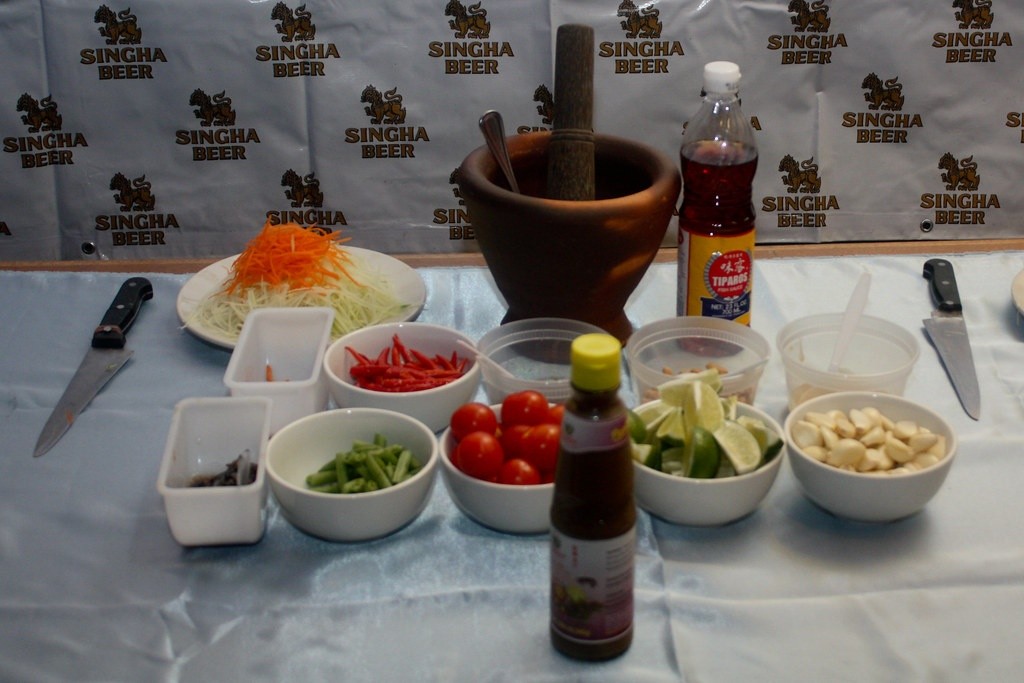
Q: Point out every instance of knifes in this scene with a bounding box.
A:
[32,277,155,456]
[921,258,980,421]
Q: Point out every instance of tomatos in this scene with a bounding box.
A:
[449,390,565,485]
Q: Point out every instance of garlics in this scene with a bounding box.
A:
[791,405,947,474]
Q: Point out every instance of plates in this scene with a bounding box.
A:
[1011,270,1023,315]
[177,246,428,351]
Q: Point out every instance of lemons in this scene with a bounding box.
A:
[624,367,786,480]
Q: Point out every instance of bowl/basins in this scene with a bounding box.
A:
[222,307,336,439]
[475,318,607,407]
[625,317,771,406]
[440,403,555,534]
[784,391,959,524]
[323,323,481,436]
[265,407,439,543]
[456,130,683,365]
[776,312,920,412]
[156,396,271,547]
[632,398,787,529]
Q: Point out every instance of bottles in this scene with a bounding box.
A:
[548,331,633,660]
[676,61,759,359]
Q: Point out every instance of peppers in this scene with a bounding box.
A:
[344,333,467,392]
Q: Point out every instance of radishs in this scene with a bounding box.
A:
[179,258,419,343]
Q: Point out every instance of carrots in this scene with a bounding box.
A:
[206,212,368,296]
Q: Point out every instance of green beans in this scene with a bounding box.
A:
[304,431,426,494]
